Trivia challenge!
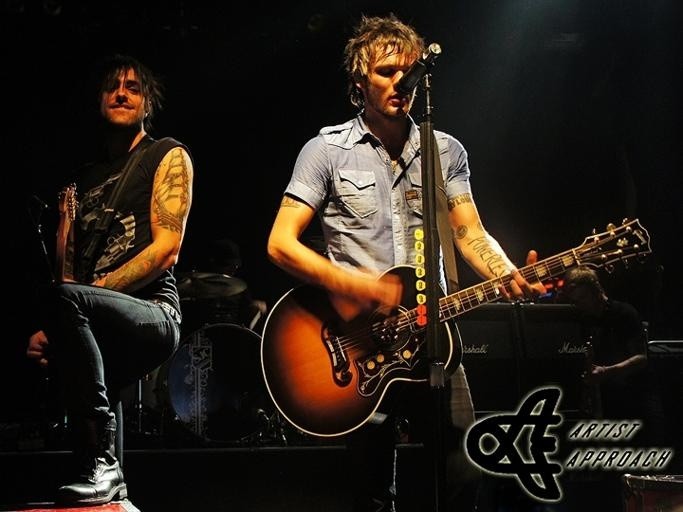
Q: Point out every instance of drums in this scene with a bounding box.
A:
[153,324,278,445]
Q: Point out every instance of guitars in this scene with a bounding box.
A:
[260,216,652,438]
[57,182,79,284]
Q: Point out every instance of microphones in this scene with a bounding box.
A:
[397,43,442,96]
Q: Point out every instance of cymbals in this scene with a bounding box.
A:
[176,273,246,300]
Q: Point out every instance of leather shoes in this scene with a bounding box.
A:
[55,450,130,505]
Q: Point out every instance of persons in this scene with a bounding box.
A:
[26,52,198,507]
[558,263,676,477]
[256,5,567,509]
[192,237,267,326]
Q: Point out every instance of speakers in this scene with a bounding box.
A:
[445,302,599,415]
